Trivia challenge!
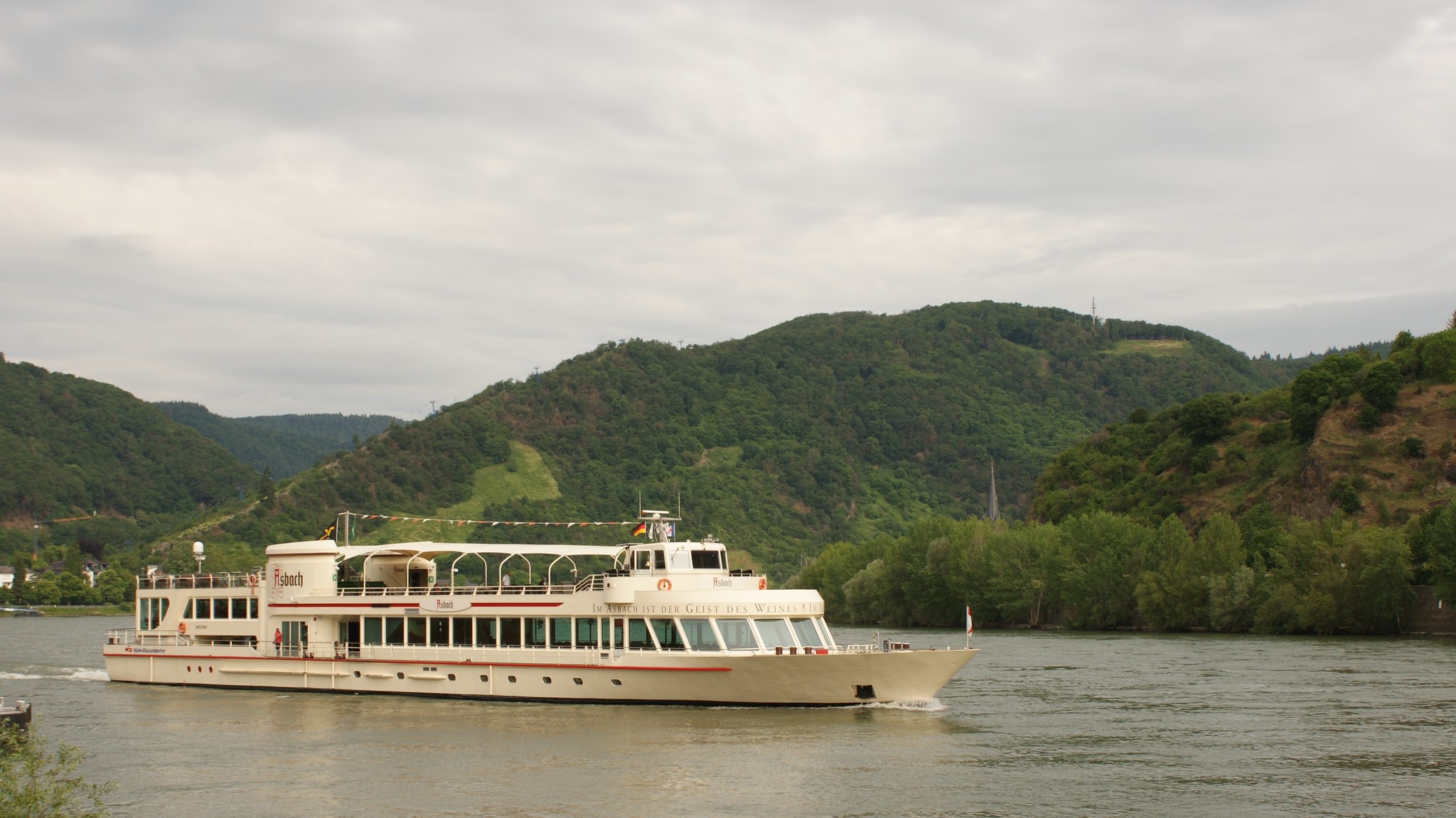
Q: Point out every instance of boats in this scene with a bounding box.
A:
[101,488,982,710]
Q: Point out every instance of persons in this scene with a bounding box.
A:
[546,583,564,594]
[535,577,548,594]
[502,571,510,594]
[273,628,282,656]
[432,583,450,595]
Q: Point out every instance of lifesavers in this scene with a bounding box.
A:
[178,622,186,635]
[657,577,672,591]
[758,577,765,591]
[248,574,256,586]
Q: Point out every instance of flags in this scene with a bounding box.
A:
[315,515,356,544]
[656,523,674,537]
[632,521,645,536]
